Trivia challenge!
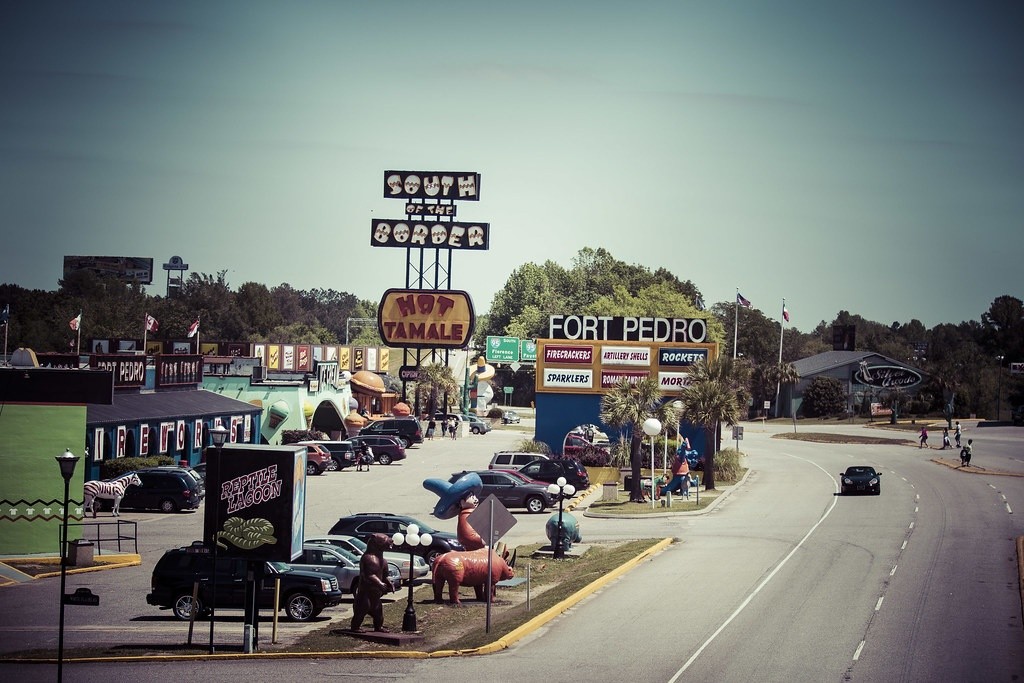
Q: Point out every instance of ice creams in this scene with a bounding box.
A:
[345,411,364,437]
[348,372,386,398]
[269,400,289,428]
[349,397,358,413]
[392,401,410,416]
[304,401,314,427]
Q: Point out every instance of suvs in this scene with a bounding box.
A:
[487,451,557,471]
[448,470,558,514]
[298,440,356,472]
[146,546,344,622]
[358,418,424,449]
[328,512,466,570]
[87,465,206,513]
[517,459,591,499]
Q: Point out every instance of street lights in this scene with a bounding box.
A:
[54,447,80,683]
[393,523,433,635]
[643,418,662,508]
[673,400,685,450]
[547,477,576,560]
[995,355,1004,420]
[209,426,231,654]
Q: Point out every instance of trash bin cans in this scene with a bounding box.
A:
[912,420,915,424]
[624,475,632,491]
[68,539,94,566]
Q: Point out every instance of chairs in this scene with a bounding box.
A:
[392,522,400,533]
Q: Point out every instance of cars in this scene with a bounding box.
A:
[302,534,431,587]
[290,544,400,600]
[564,435,610,465]
[468,416,493,435]
[423,413,470,428]
[839,466,883,495]
[344,435,407,466]
[493,469,552,487]
[501,411,520,424]
[284,443,331,475]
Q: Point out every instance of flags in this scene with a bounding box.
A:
[0,304,9,327]
[187,318,199,338]
[737,293,752,308]
[69,314,81,330]
[696,297,706,311]
[144,312,158,333]
[782,303,790,322]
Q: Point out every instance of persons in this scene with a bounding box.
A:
[960,438,973,467]
[427,417,436,440]
[441,417,458,440]
[357,440,370,472]
[954,421,961,447]
[940,427,954,450]
[918,425,929,448]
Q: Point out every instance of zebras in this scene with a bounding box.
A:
[84,469,143,519]
